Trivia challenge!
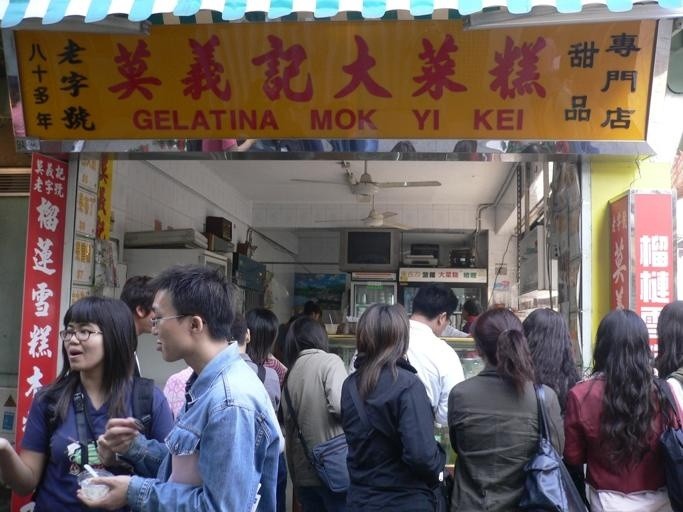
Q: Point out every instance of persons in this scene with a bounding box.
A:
[275,281,577,511]
[2,264,289,512]
[203,139,258,151]
[567,299,683,512]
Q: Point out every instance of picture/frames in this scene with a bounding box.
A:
[62,151,115,327]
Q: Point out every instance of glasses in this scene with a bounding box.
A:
[58,329,103,342]
[150,314,190,326]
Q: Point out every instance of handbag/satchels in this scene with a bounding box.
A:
[526,437,585,511]
[353,426,418,485]
[311,434,352,492]
[659,422,681,511]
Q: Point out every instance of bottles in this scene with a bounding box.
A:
[434,422,442,442]
[361,293,366,304]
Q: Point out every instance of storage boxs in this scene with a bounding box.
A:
[410,242,439,260]
[206,214,233,242]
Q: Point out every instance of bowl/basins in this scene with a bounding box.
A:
[77,468,116,502]
[324,323,339,335]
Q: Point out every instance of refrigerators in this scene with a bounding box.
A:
[124,247,229,391]
[350,271,398,321]
[399,267,488,334]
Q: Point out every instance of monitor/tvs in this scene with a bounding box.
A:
[338,228,402,273]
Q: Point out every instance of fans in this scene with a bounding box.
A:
[286,162,442,199]
[309,193,411,231]
